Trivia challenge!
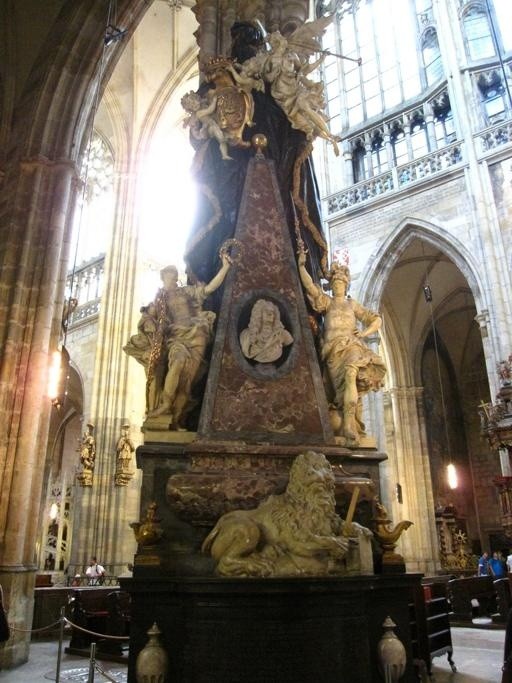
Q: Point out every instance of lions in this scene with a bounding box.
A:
[200,449,375,579]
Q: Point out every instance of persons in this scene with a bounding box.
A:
[298,247,385,447]
[178,27,345,160]
[127,252,235,433]
[239,298,294,364]
[71,556,134,585]
[477,550,512,576]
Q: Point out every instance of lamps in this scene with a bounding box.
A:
[417,238,464,493]
